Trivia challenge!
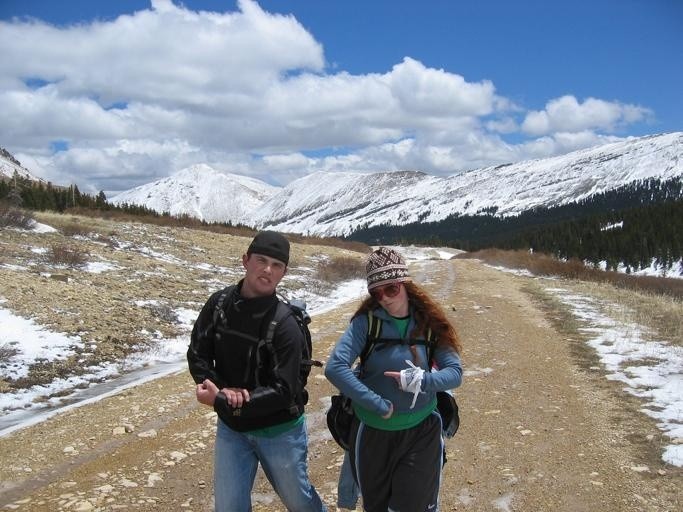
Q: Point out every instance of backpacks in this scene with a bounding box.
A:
[205,284,324,404]
[326,310,438,450]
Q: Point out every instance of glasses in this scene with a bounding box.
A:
[368,283,405,301]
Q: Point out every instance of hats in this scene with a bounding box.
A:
[366,248,412,290]
[246,232,290,264]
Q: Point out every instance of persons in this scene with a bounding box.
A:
[323,245,464,511]
[186,230,331,511]
